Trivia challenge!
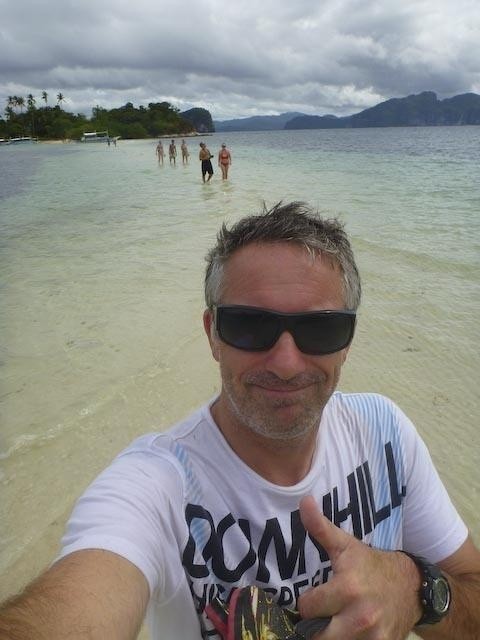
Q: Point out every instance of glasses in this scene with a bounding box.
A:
[210,302,357,357]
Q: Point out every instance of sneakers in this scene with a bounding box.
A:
[205,584,333,639]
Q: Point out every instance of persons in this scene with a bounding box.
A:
[168,139,177,164]
[218,143,231,179]
[155,139,165,164]
[181,139,190,164]
[198,141,214,183]
[0,196,480,640]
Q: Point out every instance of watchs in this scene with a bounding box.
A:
[395,549,452,628]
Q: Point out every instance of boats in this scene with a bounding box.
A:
[0,136,36,142]
[82,128,121,145]
